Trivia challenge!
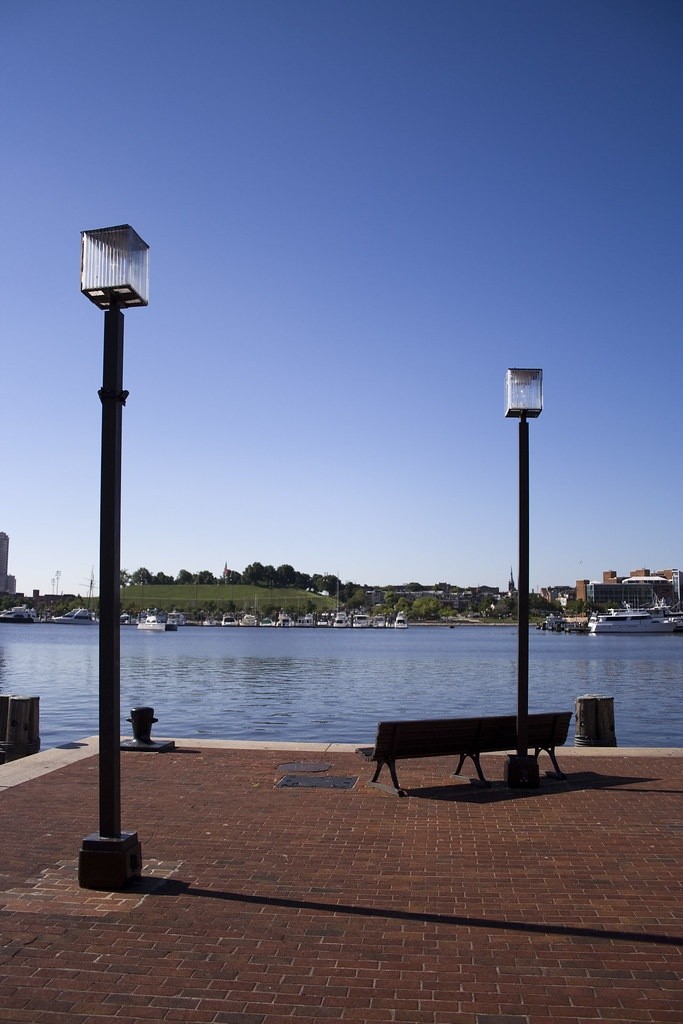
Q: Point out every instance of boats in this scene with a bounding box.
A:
[587,585,683,633]
[222,569,410,629]
[1,606,100,624]
[203,615,216,627]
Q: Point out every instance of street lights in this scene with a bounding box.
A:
[503,367,542,793]
[78,224,150,890]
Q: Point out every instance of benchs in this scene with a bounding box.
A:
[354,711,574,797]
